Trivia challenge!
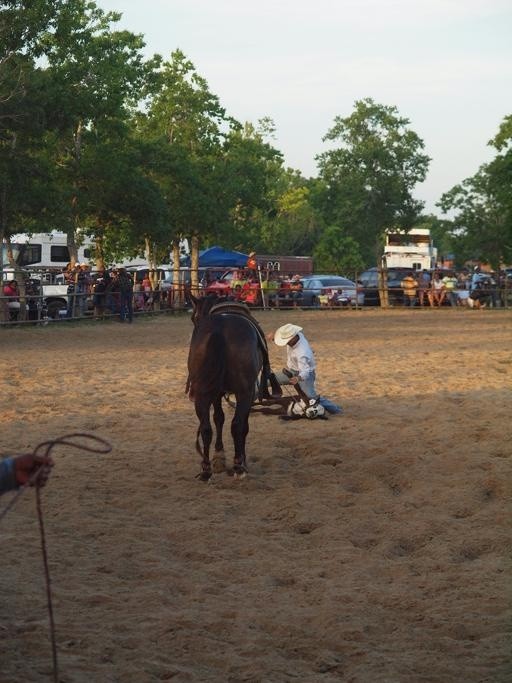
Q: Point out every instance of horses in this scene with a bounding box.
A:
[188,294,265,481]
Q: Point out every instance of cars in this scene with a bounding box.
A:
[126,266,415,309]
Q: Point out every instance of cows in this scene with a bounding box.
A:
[250,368,329,420]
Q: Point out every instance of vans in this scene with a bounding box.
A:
[3,233,190,318]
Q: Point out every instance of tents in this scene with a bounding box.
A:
[179,247,250,282]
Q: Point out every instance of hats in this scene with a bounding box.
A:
[75,262,127,272]
[274,323,303,347]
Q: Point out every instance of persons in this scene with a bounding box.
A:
[231,270,260,306]
[262,272,303,307]
[184,280,191,303]
[247,252,258,278]
[162,280,173,297]
[259,323,342,416]
[0,450,55,498]
[401,265,506,308]
[3,280,19,322]
[64,262,133,323]
[317,287,351,310]
[132,272,151,311]
